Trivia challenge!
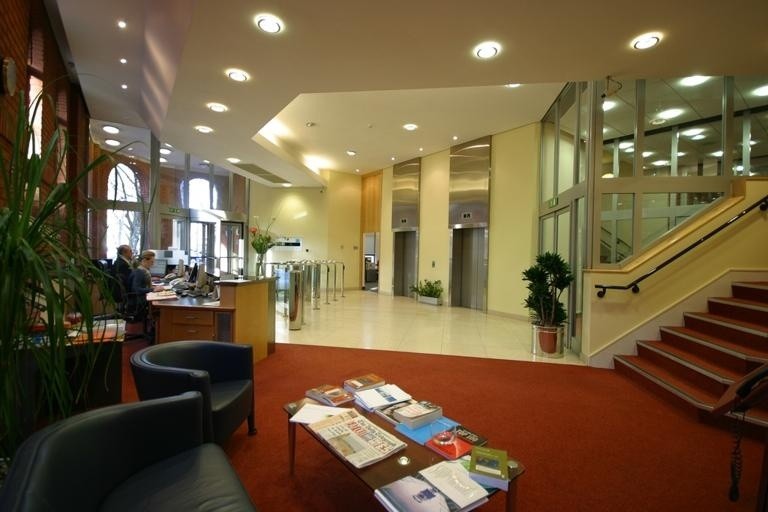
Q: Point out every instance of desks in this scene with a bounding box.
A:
[149,276,236,344]
[281,378,527,512]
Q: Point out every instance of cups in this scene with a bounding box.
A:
[431,423,456,446]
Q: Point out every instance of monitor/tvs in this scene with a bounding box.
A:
[148,259,167,277]
[188,262,198,282]
[194,263,205,291]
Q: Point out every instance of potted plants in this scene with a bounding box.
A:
[249,215,279,279]
[407,279,443,305]
[521,251,575,358]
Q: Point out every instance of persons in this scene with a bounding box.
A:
[130,251,155,346]
[112,245,136,299]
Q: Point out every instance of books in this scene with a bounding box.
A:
[290,373,508,512]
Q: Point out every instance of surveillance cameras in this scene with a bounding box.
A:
[601,86,618,99]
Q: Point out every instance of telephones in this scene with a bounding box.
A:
[735,368,768,398]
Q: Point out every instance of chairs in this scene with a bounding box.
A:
[0,390,258,512]
[130,340,257,445]
[117,274,152,344]
[94,259,125,320]
